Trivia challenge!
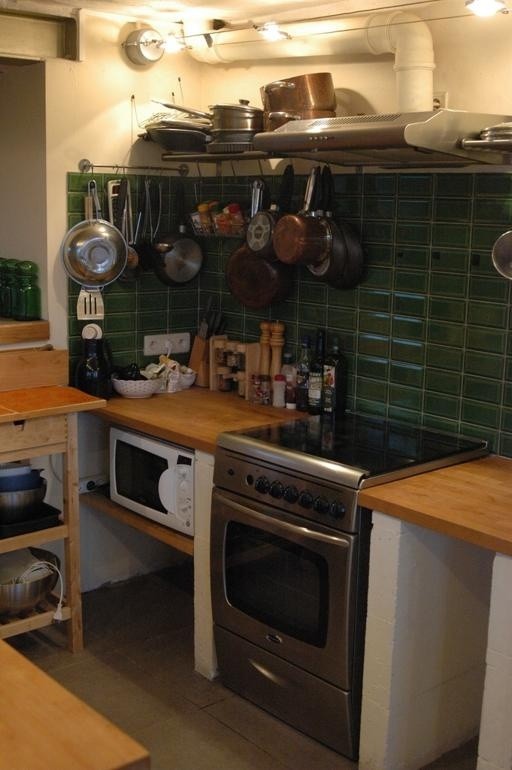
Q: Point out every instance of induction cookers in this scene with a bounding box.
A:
[215,403,493,491]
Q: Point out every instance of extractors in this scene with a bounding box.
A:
[258,108,511,171]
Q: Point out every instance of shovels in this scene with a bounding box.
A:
[77,179,105,320]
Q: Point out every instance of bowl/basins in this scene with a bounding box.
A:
[1,477,49,527]
[62,217,125,290]
[259,71,338,112]
[180,371,197,391]
[0,547,61,613]
[107,374,167,401]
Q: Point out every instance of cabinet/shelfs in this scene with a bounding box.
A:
[0,386,107,653]
[79,386,310,557]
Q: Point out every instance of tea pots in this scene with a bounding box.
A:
[77,338,114,401]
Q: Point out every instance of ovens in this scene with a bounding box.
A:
[208,485,368,764]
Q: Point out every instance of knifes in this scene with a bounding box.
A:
[197,294,226,341]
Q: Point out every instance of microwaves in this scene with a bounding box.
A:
[109,426,197,538]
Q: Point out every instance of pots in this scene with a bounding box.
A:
[261,106,337,133]
[224,174,291,308]
[153,164,203,289]
[208,100,267,133]
[271,166,332,265]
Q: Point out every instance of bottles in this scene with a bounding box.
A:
[250,374,272,404]
[285,376,297,409]
[295,326,349,416]
[0,256,41,325]
[273,375,285,408]
[280,352,296,382]
